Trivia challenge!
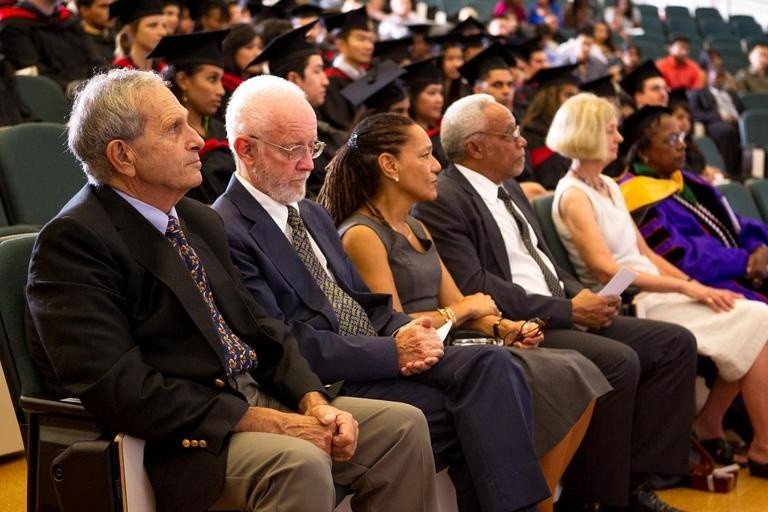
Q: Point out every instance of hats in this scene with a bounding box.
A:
[103,1,664,113]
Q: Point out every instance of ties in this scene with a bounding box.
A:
[162,214,261,375]
[494,186,570,302]
[282,201,379,342]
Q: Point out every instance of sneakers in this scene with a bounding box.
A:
[694,424,768,482]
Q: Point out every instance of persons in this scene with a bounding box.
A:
[0,0,767,512]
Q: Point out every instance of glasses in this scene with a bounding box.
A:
[462,124,524,142]
[653,135,681,148]
[249,128,327,164]
